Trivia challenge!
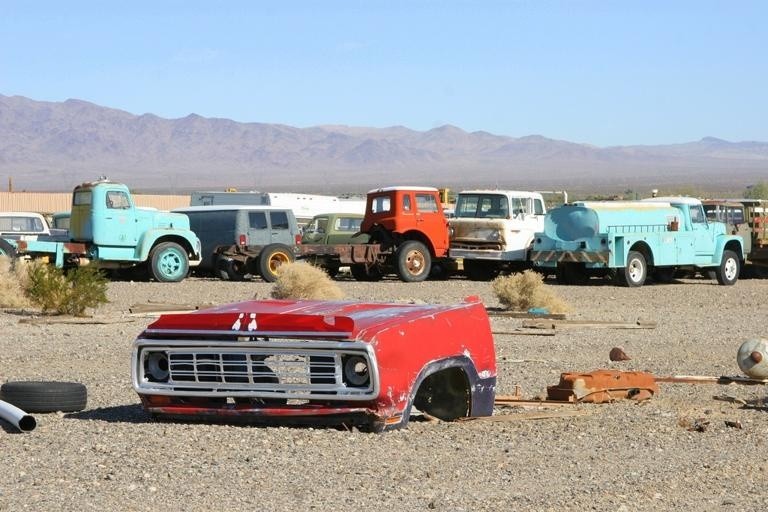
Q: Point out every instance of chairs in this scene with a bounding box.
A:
[487,200,505,218]
[31,218,43,232]
[107,194,113,208]
[346,219,356,232]
[403,196,411,210]
